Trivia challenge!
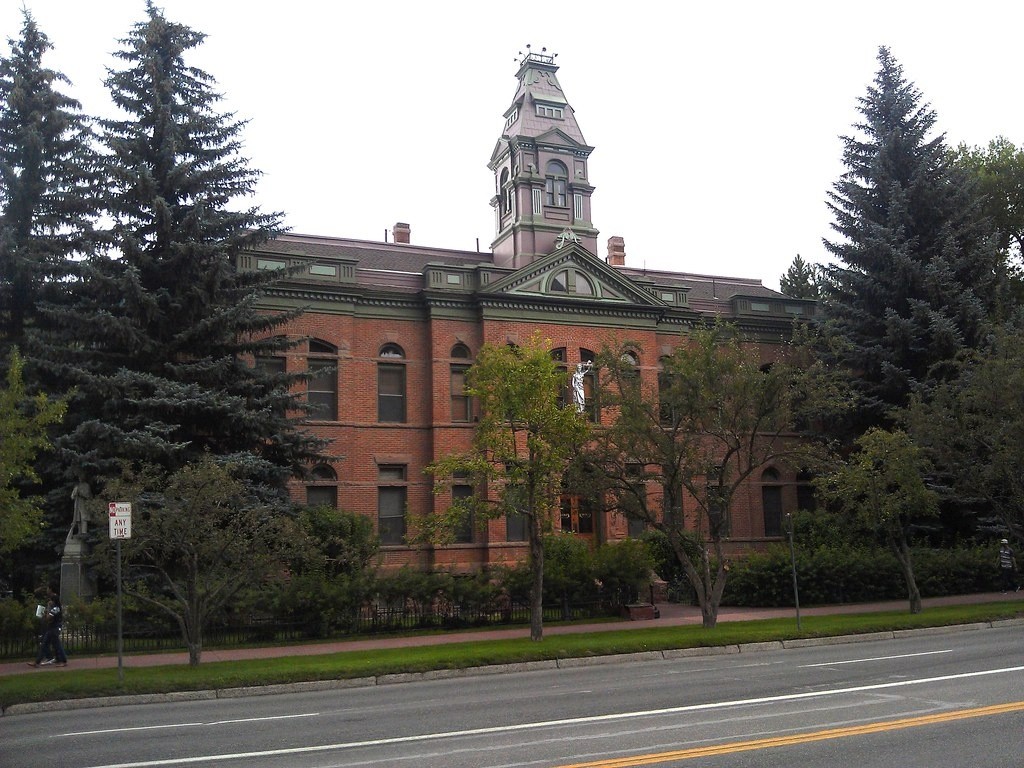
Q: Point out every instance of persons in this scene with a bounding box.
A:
[27,584,67,667]
[996,539,1020,594]
[71,473,92,534]
[572,363,588,411]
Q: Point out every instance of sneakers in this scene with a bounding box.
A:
[27,660,40,668]
[1000,590,1008,594]
[42,658,55,665]
[55,661,69,666]
[1014,586,1020,593]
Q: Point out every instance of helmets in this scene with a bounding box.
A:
[1001,538,1008,544]
